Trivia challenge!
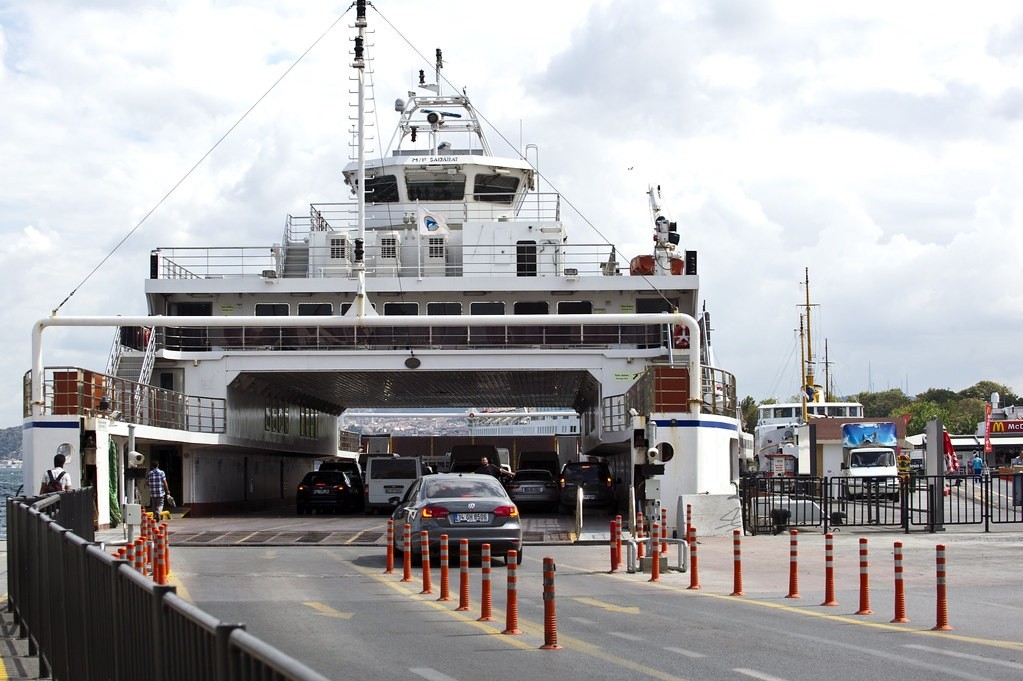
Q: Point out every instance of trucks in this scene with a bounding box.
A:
[358,453,400,473]
[840,422,900,502]
[497,448,511,472]
[450,445,500,479]
[516,451,560,482]
[364,456,422,515]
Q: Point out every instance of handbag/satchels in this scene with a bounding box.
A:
[167,494,175,508]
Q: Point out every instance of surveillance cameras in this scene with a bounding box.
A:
[647,448,658,459]
[129,450,144,464]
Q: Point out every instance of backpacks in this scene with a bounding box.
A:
[45,470,66,493]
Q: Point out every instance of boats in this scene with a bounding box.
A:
[754,267,864,492]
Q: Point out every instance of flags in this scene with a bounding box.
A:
[984,405,992,452]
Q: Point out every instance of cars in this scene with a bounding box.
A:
[506,469,560,514]
[388,473,524,565]
[297,470,356,517]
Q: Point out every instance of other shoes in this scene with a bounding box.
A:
[155,512,159,523]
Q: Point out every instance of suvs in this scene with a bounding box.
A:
[558,459,615,515]
[319,460,364,511]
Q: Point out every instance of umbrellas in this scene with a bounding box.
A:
[943,425,959,475]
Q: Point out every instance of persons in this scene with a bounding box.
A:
[470,457,515,478]
[40,454,70,495]
[1013,451,1023,465]
[145,461,171,523]
[969,454,983,483]
